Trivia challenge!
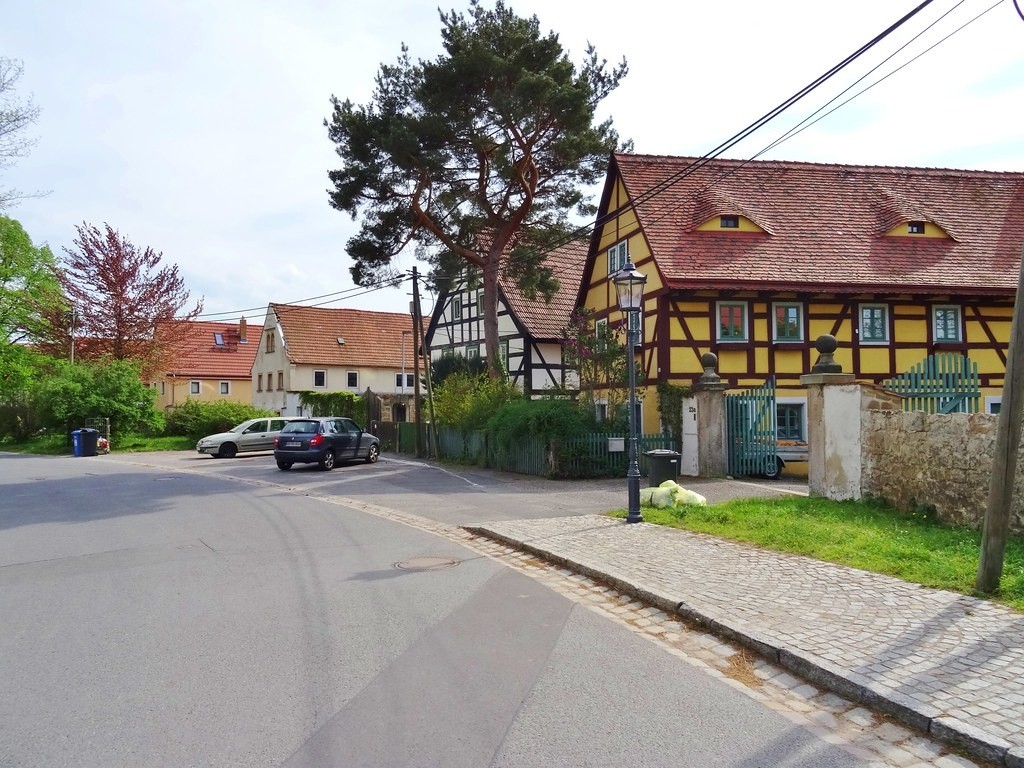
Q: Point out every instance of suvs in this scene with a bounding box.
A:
[274,416,382,472]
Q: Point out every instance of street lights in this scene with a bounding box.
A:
[401,330,413,395]
[612,252,649,522]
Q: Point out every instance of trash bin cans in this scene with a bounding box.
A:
[79,430,99,457]
[71,430,82,457]
[641,449,682,487]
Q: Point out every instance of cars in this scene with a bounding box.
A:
[196,417,300,459]
